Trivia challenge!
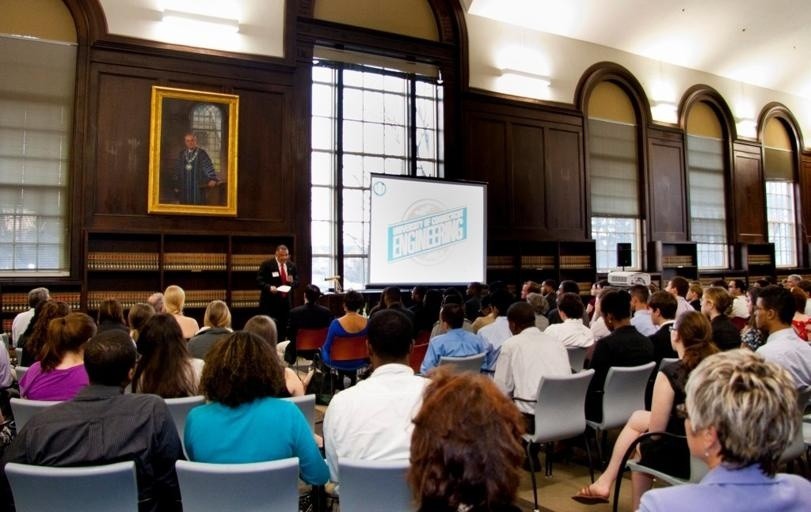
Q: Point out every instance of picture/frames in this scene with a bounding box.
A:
[147,85,241,219]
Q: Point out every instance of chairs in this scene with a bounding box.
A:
[659,357,677,371]
[408,341,429,374]
[584,361,656,483]
[299,328,328,377]
[286,392,316,439]
[158,396,204,452]
[515,366,594,510]
[611,420,710,510]
[5,461,142,510]
[329,337,371,397]
[175,457,302,510]
[773,384,809,479]
[335,457,418,511]
[437,348,485,377]
[0,323,29,389]
[566,337,592,373]
[10,398,65,432]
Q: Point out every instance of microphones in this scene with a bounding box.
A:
[325,276,340,281]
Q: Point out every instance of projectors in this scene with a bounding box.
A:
[607,272,651,287]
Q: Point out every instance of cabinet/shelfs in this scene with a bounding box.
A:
[483,235,779,289]
[82,227,163,317]
[229,235,295,334]
[165,231,230,328]
[0,278,86,335]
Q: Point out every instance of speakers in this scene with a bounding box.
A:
[617,243,631,266]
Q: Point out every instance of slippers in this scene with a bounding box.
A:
[571,485,610,505]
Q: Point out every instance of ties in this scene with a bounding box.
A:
[280,263,288,297]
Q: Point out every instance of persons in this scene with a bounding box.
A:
[1,273,811,512]
[256,245,303,343]
[175,132,219,206]
